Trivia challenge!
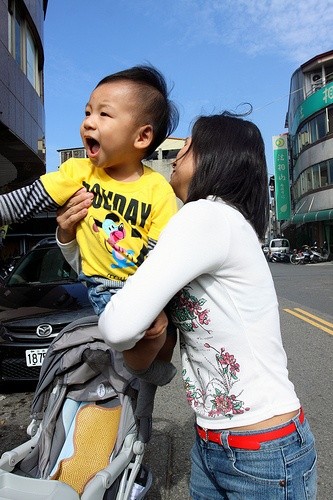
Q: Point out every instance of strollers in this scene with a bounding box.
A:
[1,316,153,500]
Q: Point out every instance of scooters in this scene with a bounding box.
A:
[266,240,331,264]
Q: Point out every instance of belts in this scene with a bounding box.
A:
[197,408,305,450]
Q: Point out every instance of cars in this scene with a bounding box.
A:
[263,247,269,254]
[0,236,102,383]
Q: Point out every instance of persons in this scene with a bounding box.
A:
[55,102,316,500]
[0,66,179,420]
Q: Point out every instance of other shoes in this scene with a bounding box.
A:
[123,360,177,386]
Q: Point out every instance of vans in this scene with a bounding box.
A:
[269,238,290,262]
